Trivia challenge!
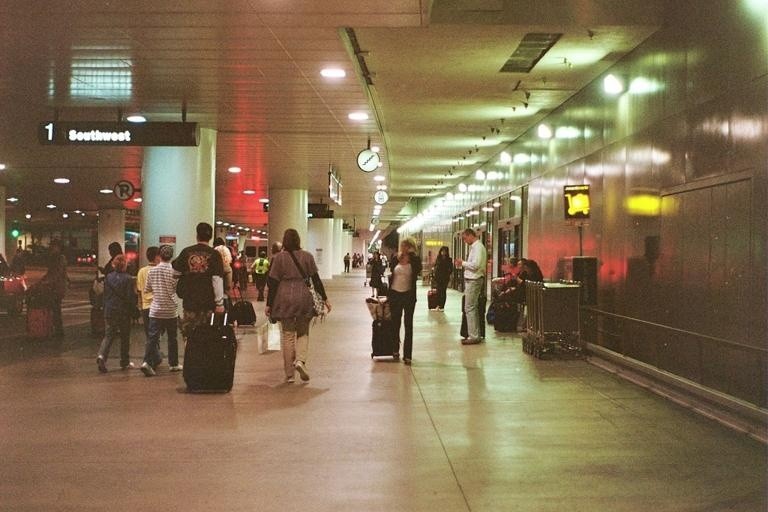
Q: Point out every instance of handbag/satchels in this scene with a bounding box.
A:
[287,250,328,317]
[105,277,140,319]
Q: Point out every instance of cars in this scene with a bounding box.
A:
[0,253,27,316]
[77,249,97,265]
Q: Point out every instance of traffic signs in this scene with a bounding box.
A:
[114,179,135,201]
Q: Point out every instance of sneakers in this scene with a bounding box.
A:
[140,362,157,376]
[404,358,410,364]
[96,355,107,372]
[287,377,294,382]
[295,361,310,382]
[393,354,400,360]
[169,364,183,371]
[461,339,480,344]
[121,362,135,369]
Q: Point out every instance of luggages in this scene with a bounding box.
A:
[27,294,55,341]
[377,276,387,296]
[232,287,257,327]
[184,310,238,392]
[90,306,106,339]
[495,272,518,331]
[461,291,486,340]
[371,301,393,359]
[428,288,438,310]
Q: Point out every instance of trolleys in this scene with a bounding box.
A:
[521,278,582,359]
[364,263,373,285]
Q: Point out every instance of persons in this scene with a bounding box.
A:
[455,229,486,345]
[491,257,544,330]
[237,251,248,291]
[171,223,228,336]
[343,252,364,273]
[387,236,423,366]
[367,251,384,300]
[139,245,183,377]
[431,246,453,311]
[250,251,270,301]
[39,240,70,333]
[96,242,123,278]
[137,247,161,339]
[213,237,233,304]
[96,254,135,373]
[380,251,387,277]
[265,228,331,383]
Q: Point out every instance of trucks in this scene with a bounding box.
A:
[242,240,267,274]
